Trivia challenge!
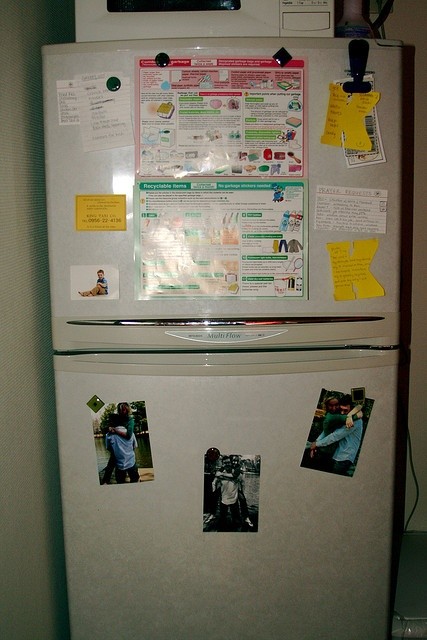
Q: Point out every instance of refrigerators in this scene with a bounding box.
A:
[36,39,404,637]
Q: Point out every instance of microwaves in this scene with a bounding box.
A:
[74,0,336,42]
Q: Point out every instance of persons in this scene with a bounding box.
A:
[78,269,108,296]
[105,415,140,484]
[101,402,135,484]
[310,394,363,475]
[312,395,363,471]
[212,456,242,527]
[205,455,255,527]
